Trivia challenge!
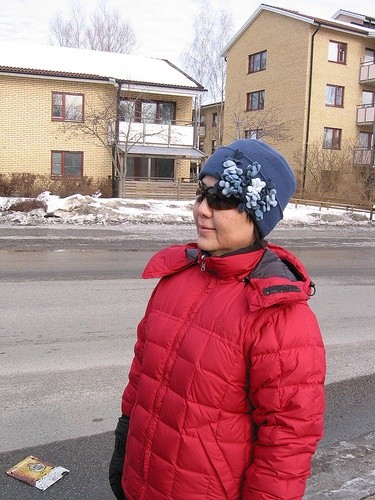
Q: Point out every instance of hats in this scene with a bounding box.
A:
[196,138,296,241]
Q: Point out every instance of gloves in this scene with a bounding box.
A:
[108,414,131,500]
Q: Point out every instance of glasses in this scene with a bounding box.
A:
[195,180,241,211]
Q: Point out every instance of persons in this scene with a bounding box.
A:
[108,139,326,500]
